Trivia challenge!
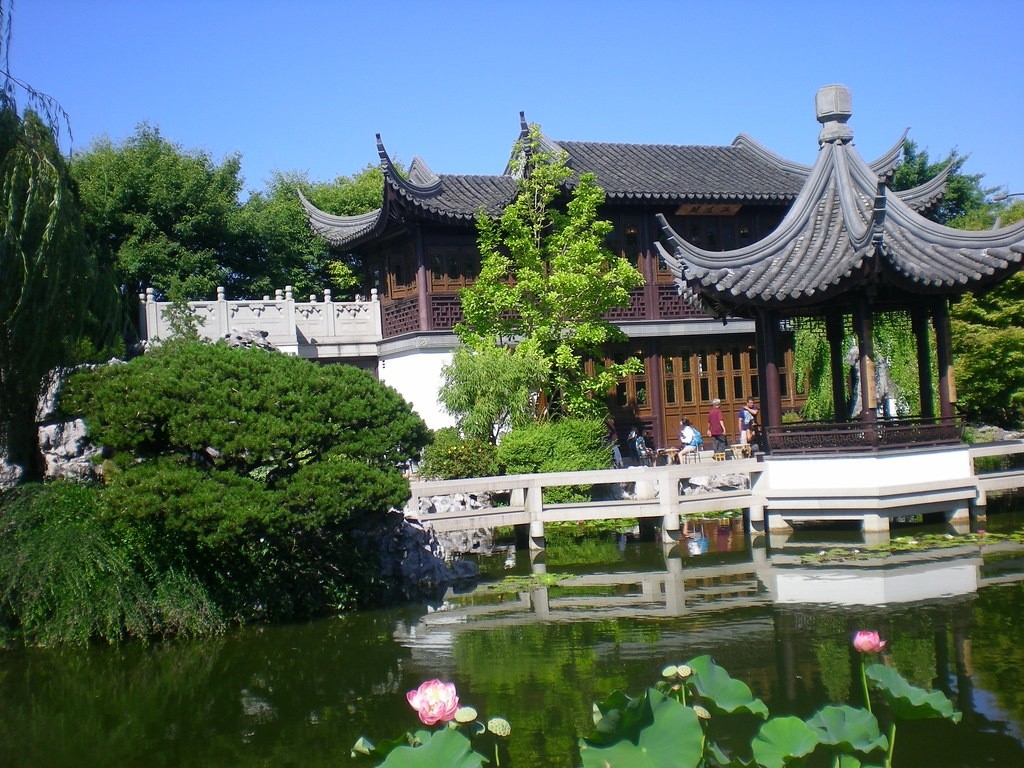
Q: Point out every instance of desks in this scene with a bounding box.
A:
[658,448,679,465]
[730,444,752,459]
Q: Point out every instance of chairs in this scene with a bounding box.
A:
[684,442,701,465]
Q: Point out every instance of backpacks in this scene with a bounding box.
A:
[690,425,703,452]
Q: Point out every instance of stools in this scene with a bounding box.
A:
[715,452,726,461]
[639,455,653,467]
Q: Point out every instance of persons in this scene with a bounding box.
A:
[746,419,760,452]
[627,426,656,467]
[677,417,696,465]
[738,396,759,451]
[606,419,624,468]
[707,399,728,460]
